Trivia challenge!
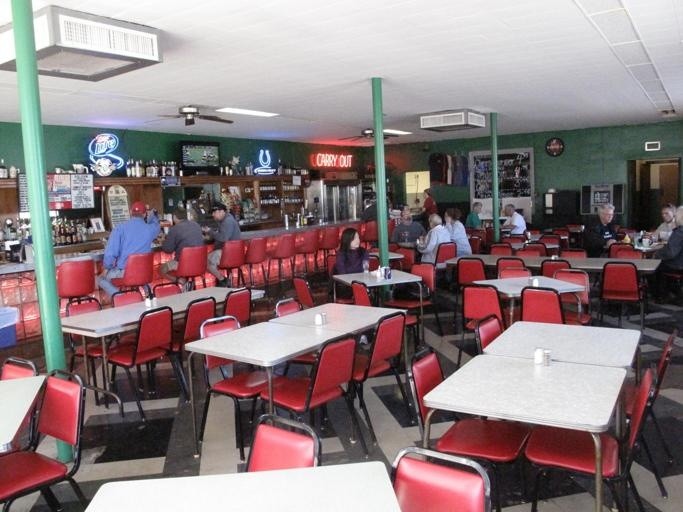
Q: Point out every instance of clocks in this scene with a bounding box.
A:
[546,138,566,159]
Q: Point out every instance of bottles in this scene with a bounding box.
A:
[218,159,253,176]
[124,158,183,177]
[534,348,551,367]
[0,158,8,178]
[50,215,87,246]
[275,158,295,175]
[315,312,326,326]
[283,191,303,203]
[260,183,280,207]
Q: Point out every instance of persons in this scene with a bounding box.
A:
[204,202,241,289]
[332,226,369,298]
[361,198,380,248]
[159,205,207,292]
[389,188,683,305]
[97,201,162,304]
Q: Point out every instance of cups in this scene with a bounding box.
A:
[641,238,652,247]
[363,260,369,273]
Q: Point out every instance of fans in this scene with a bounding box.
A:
[144,106,236,129]
[339,127,402,145]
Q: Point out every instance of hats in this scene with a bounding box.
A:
[209,202,226,211]
[132,202,145,214]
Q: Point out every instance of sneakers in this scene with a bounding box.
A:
[655,295,671,305]
[182,282,193,293]
[360,335,367,344]
[215,276,231,288]
[144,291,153,300]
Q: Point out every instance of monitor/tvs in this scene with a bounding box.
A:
[179,140,220,175]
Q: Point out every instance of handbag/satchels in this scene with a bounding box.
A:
[392,282,430,301]
